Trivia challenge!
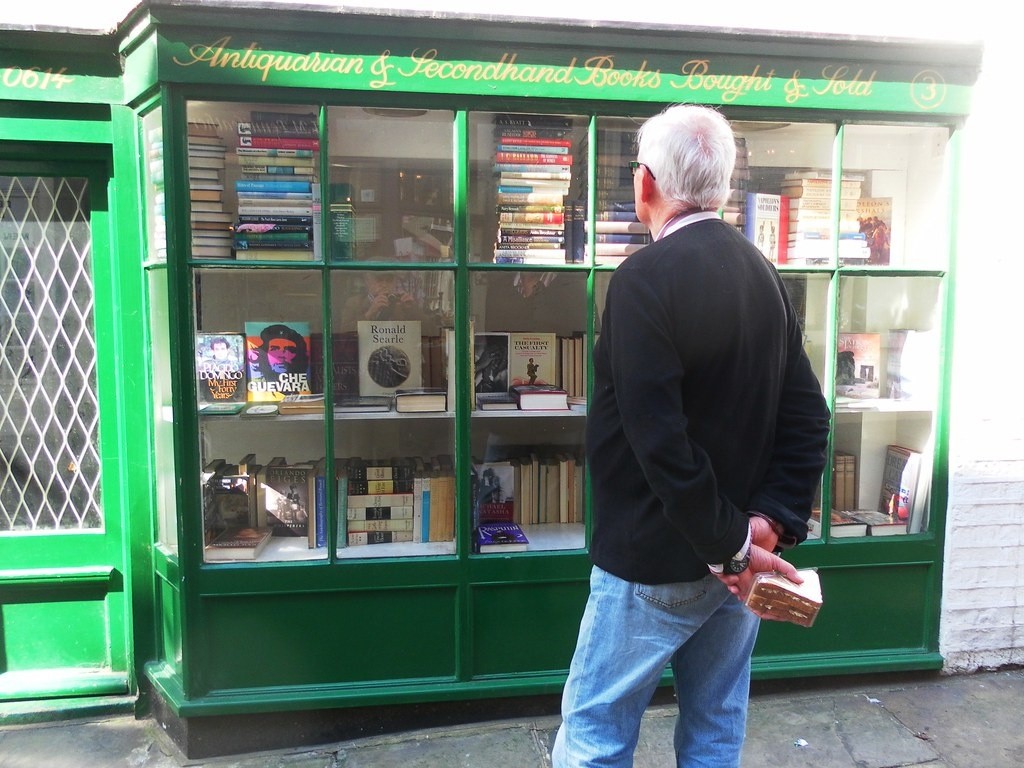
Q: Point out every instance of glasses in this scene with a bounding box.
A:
[629,161,655,180]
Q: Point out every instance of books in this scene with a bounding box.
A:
[474,330,600,411]
[838,333,881,399]
[147,112,355,259]
[200,453,455,561]
[195,321,455,416]
[471,451,585,554]
[491,115,650,265]
[808,445,930,538]
[722,138,893,266]
[886,328,924,400]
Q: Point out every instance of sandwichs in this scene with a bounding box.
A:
[745,569,823,628]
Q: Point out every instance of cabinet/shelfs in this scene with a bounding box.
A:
[106,0,986,758]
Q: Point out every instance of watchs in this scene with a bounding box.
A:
[724,546,751,575]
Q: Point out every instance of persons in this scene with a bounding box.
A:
[340,257,437,338]
[551,108,831,768]
[277,490,286,519]
[201,338,235,372]
[484,272,601,333]
[526,358,537,384]
[284,484,300,521]
[492,529,509,543]
